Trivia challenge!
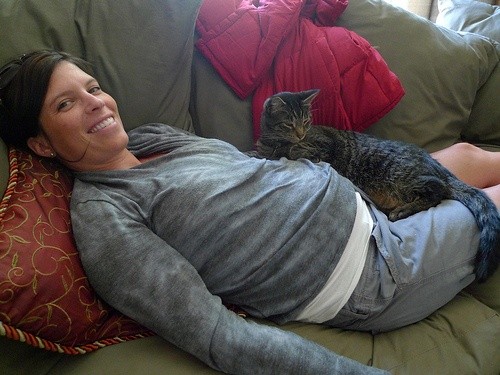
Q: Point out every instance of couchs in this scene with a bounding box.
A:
[2,2,500,373]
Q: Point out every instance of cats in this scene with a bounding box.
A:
[243,88,500,287]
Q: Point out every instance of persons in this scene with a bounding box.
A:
[2,49,500,375]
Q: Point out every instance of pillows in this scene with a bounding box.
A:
[0,146,159,354]
